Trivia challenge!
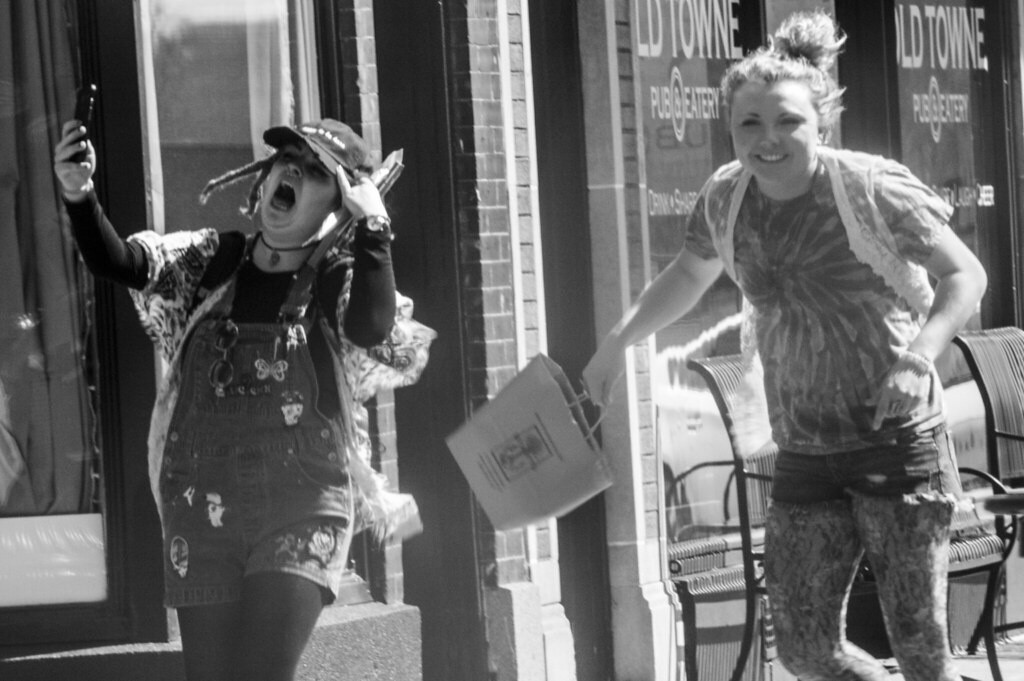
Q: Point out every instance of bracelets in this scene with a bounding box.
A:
[898,350,931,369]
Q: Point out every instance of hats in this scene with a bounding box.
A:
[262,118,375,185]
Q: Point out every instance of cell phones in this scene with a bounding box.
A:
[67,84,96,161]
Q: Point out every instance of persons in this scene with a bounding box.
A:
[54,120,397,681]
[582,14,987,681]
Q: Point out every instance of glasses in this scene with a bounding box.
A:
[207,316,240,398]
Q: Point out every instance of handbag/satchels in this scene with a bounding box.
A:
[445,352,615,531]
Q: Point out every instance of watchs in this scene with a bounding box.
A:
[353,215,391,231]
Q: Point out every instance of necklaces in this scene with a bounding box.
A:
[260,232,316,266]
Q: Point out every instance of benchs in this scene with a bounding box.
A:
[951,326,1024,656]
[686,353,1019,681]
